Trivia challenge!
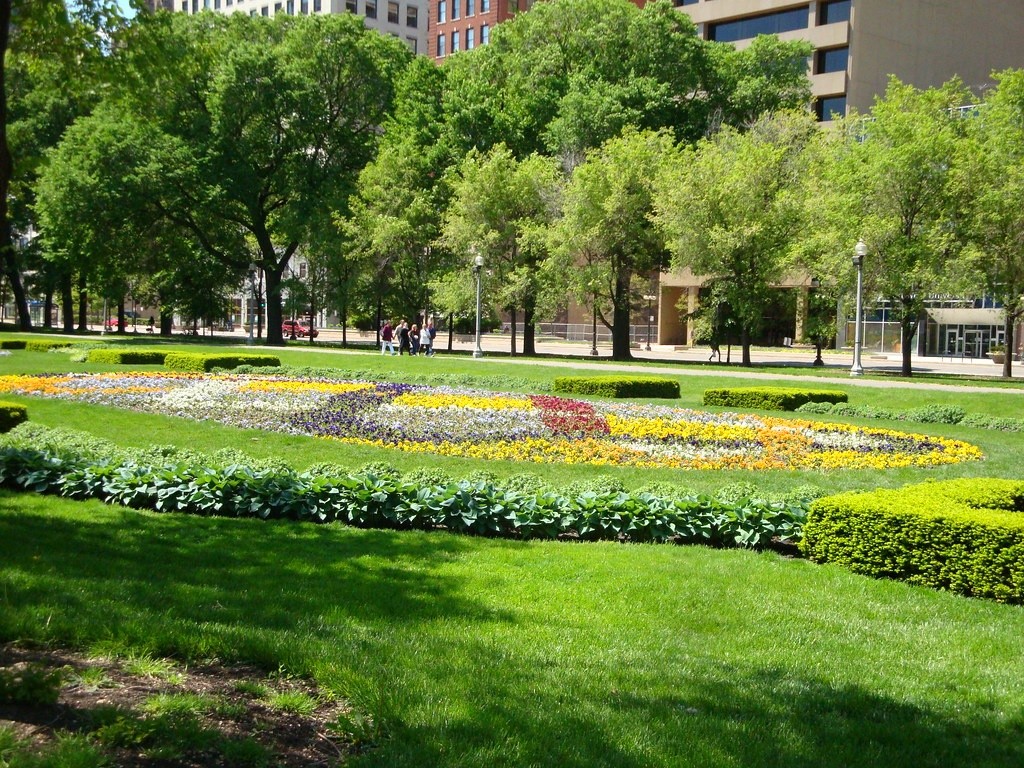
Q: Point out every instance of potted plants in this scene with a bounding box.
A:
[986,346,1017,364]
[243,321,254,332]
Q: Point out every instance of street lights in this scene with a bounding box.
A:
[643,288,656,352]
[849,238,868,377]
[471,252,484,359]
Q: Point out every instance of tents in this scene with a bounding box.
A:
[12,299,59,328]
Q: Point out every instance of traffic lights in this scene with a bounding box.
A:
[261,299,265,307]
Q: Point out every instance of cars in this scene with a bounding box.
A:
[102,317,129,327]
[282,319,320,337]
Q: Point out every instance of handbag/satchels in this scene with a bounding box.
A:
[379,331,384,336]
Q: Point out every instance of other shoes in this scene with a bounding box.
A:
[430,355,434,357]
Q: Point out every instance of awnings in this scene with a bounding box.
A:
[923,307,1006,325]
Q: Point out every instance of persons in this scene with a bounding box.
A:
[394,319,405,356]
[504,325,509,334]
[148,316,155,334]
[419,323,431,356]
[379,324,387,337]
[225,319,232,332]
[399,322,412,356]
[408,324,420,357]
[428,322,436,357]
[382,320,396,356]
